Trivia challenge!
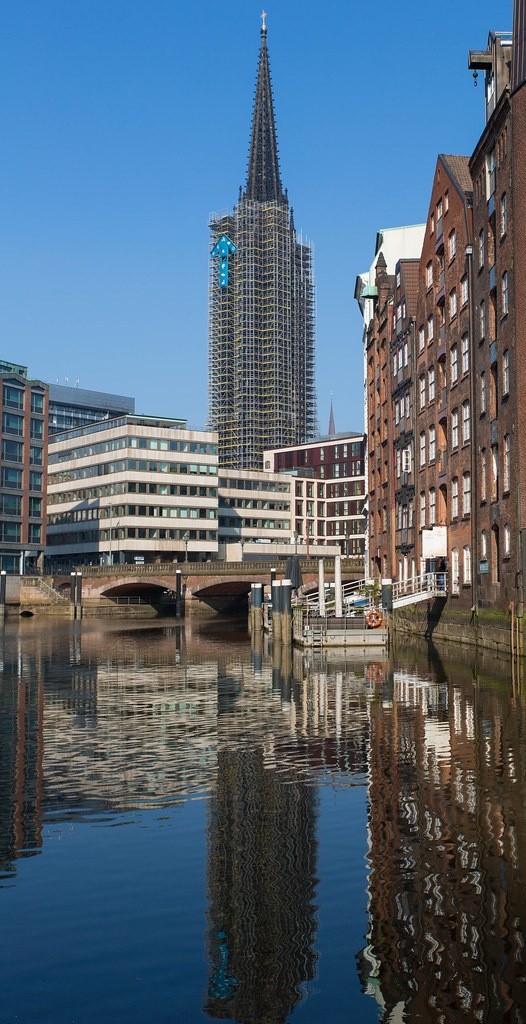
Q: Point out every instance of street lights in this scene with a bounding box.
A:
[292,530,299,558]
[184,533,191,565]
[345,532,350,559]
[104,501,112,565]
[240,537,245,562]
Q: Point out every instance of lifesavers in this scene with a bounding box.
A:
[366,610,383,628]
